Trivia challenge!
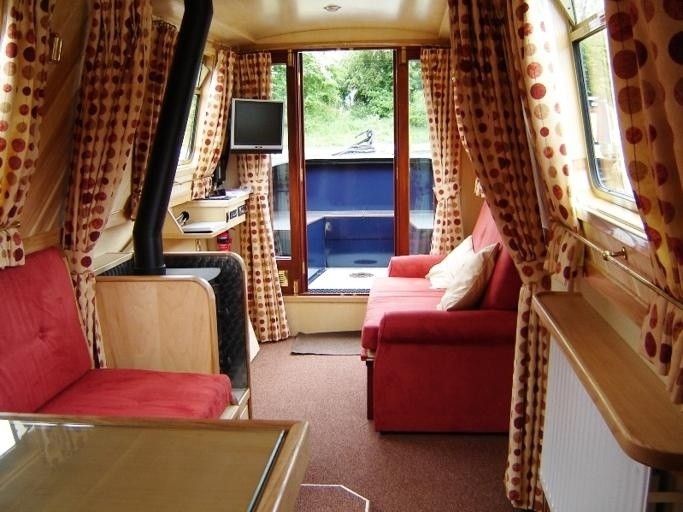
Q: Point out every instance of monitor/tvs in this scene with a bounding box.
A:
[231,98,283,150]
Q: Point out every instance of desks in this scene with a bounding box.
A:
[0,421,309,511]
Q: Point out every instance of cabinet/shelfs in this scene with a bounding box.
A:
[159,190,249,239]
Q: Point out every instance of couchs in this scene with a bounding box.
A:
[0,245,234,417]
[360,198,540,435]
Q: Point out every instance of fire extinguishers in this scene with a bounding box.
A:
[216,228,236,251]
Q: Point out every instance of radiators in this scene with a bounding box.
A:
[541,333,651,510]
